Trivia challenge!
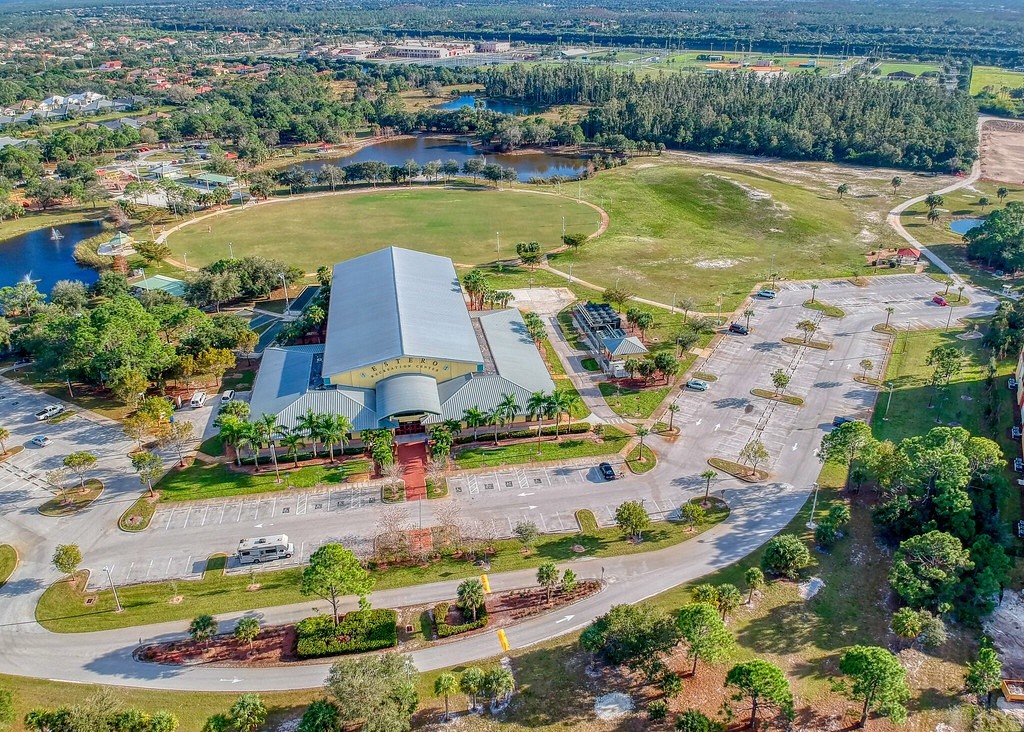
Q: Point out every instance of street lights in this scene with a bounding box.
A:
[809,482,819,527]
[139,392,145,403]
[677,337,682,356]
[279,273,291,315]
[885,383,893,418]
[103,566,121,610]
[719,293,725,324]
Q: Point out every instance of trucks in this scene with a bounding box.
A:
[237,534,294,564]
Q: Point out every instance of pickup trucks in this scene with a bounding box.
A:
[35,403,65,421]
[833,416,857,427]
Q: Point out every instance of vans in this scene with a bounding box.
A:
[190,392,207,408]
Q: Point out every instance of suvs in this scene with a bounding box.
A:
[221,389,235,404]
[758,290,776,299]
[729,324,749,335]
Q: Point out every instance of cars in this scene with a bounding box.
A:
[32,435,52,447]
[933,295,947,306]
[599,462,616,480]
[687,380,710,391]
